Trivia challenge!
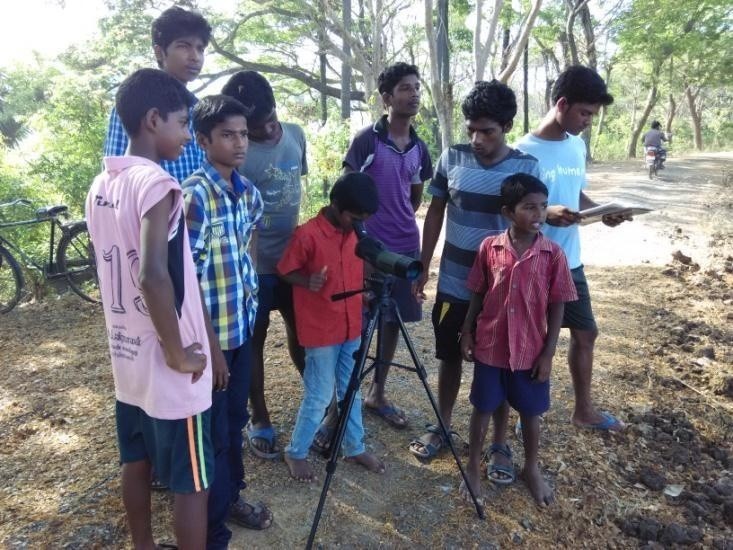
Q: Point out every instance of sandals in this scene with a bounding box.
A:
[408,426,448,459]
[485,442,516,485]
[224,496,273,530]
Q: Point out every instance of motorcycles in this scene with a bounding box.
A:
[644,134,672,181]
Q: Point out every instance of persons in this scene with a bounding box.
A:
[312,64,433,452]
[641,123,667,169]
[458,173,578,508]
[106,8,212,490]
[84,67,214,550]
[222,70,306,459]
[279,173,386,482]
[408,81,541,484]
[182,97,273,528]
[515,65,632,442]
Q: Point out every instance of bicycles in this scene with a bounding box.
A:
[0,199,103,314]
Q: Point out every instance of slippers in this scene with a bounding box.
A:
[312,424,337,453]
[569,412,623,433]
[363,401,410,430]
[245,424,282,459]
[514,415,526,443]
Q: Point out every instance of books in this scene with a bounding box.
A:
[578,203,654,226]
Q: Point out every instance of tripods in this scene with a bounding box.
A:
[304,272,485,550]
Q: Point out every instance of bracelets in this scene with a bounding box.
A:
[458,331,472,337]
